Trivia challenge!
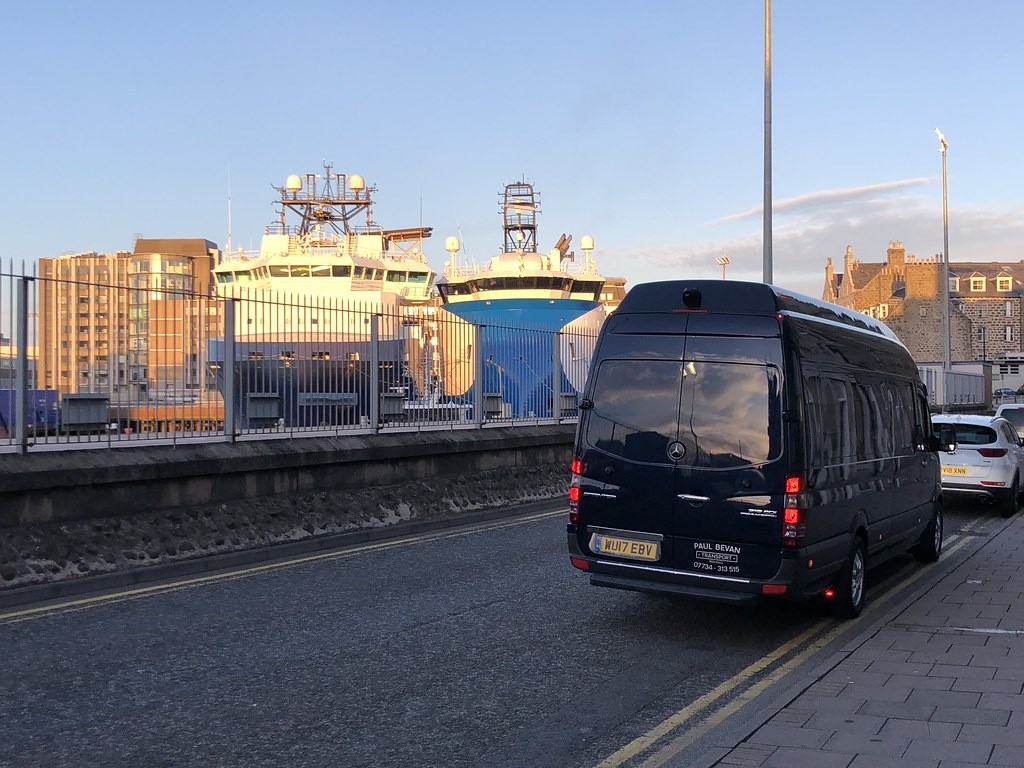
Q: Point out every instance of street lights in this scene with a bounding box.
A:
[715,256,732,281]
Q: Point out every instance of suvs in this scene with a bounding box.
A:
[928,411,1024,517]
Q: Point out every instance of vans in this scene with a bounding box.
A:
[567,279,949,621]
[994,403,1024,447]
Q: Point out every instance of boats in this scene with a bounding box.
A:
[203,159,440,431]
